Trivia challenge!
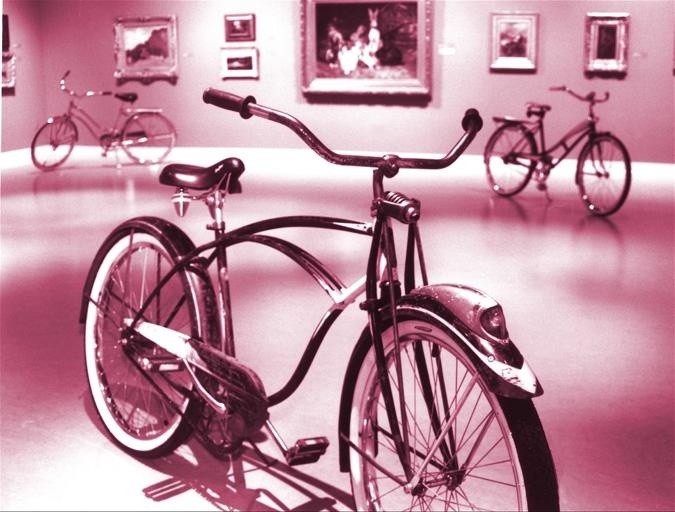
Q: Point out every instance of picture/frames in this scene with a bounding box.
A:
[224,14,255,42]
[584,13,631,73]
[299,0,434,102]
[2,52,16,89]
[488,11,540,70]
[220,46,259,79]
[112,14,178,80]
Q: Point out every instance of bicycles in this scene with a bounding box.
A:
[26,72,178,174]
[76,87,563,512]
[485,84,630,220]
